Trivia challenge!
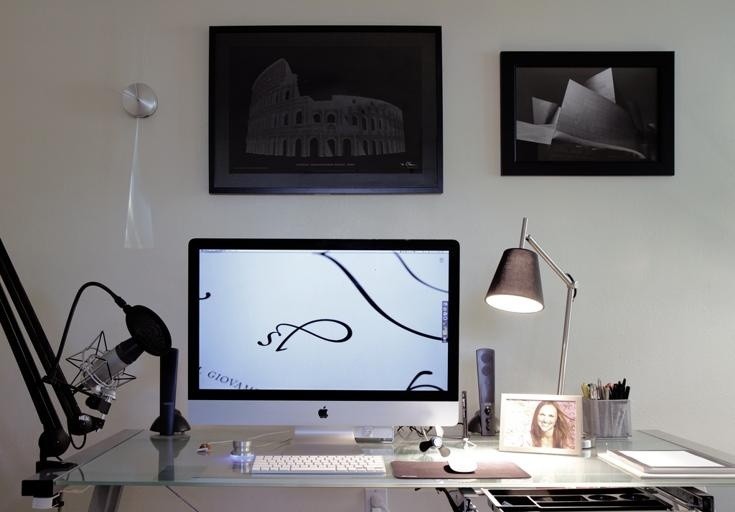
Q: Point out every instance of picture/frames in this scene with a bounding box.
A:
[209,24,443,194]
[501,50,675,177]
[498,393,583,456]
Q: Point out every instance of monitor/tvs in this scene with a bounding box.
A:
[188,238,461,456]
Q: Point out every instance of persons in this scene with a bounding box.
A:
[519,399,574,451]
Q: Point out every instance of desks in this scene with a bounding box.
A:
[55,423,734,512]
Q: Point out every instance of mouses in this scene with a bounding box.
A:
[447,452,478,474]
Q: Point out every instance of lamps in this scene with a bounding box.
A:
[484,217,597,450]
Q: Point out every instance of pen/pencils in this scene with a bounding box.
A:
[581,378,630,438]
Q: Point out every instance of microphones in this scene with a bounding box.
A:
[79,304,172,389]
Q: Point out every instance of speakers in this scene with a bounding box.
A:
[469,348,499,437]
[150,349,191,436]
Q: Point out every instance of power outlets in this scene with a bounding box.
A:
[365,489,389,512]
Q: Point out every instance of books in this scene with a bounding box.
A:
[597,451,734,485]
[606,449,735,473]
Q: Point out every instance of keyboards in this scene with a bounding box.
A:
[251,454,386,475]
